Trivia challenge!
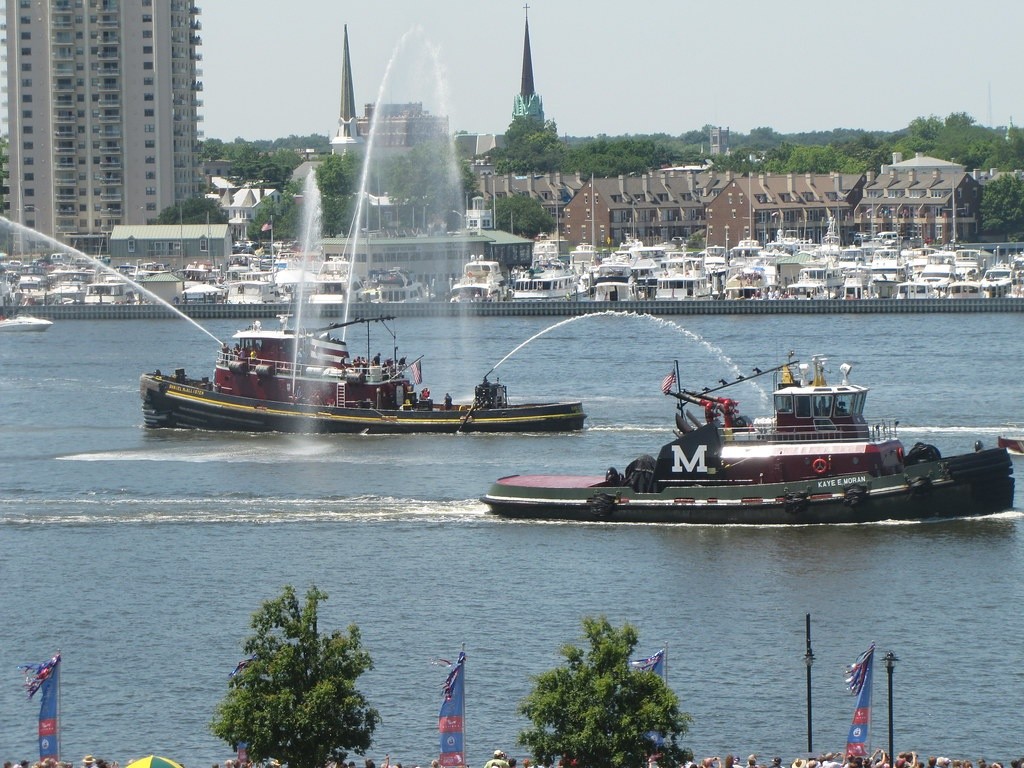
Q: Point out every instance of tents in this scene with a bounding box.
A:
[182,283,225,303]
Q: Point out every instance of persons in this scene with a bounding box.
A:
[352,353,407,379]
[643,748,1024,768]
[3,756,284,768]
[221,342,258,365]
[298,348,307,375]
[339,354,346,381]
[423,388,429,399]
[443,392,453,411]
[325,749,577,768]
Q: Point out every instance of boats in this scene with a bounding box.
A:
[477,349,1016,525]
[140,314,589,436]
[997,421,1024,454]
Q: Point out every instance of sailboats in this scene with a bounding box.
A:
[0,174,1024,305]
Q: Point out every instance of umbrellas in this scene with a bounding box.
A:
[126,754,183,768]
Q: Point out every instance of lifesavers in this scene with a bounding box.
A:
[898,447,903,462]
[813,458,827,474]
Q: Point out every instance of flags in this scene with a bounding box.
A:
[659,369,676,395]
[261,221,272,231]
[410,359,423,385]
